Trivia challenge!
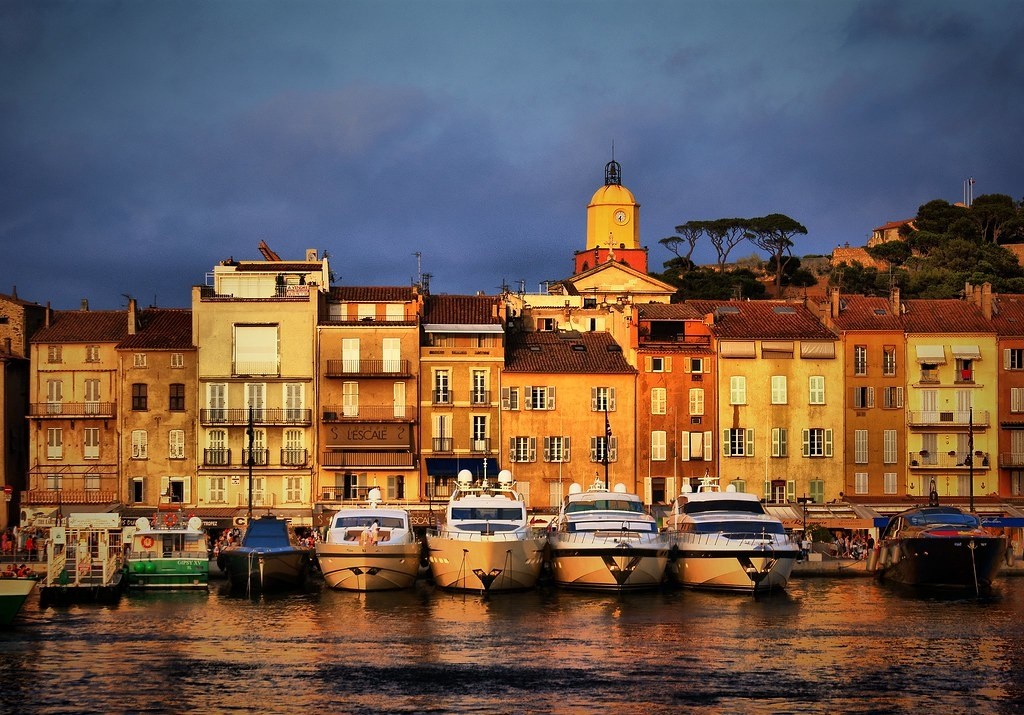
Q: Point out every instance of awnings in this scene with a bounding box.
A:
[47,501,447,527]
[916,345,947,365]
[762,504,894,530]
[426,457,500,478]
[951,345,982,360]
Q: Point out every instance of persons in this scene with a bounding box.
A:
[368,519,380,546]
[287,519,324,550]
[0,513,49,563]
[54,511,243,589]
[998,531,1016,567]
[0,562,38,580]
[368,486,382,509]
[754,530,877,559]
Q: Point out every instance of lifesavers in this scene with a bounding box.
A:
[140,536,154,548]
[152,513,157,525]
[165,513,177,527]
[186,511,195,520]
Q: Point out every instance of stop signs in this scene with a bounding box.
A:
[4,485,12,494]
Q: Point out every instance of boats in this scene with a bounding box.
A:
[663,474,802,595]
[866,478,1015,599]
[122,511,212,591]
[216,510,317,593]
[551,472,674,597]
[425,457,549,596]
[314,487,423,593]
[0,576,41,632]
